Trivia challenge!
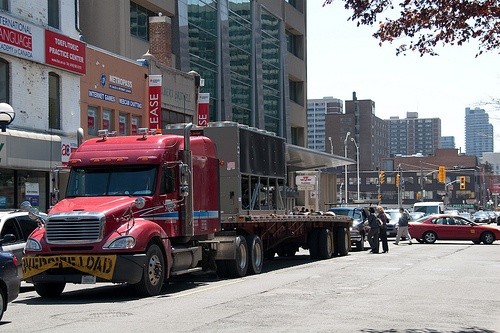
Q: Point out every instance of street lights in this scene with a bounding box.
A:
[344,132,351,204]
[351,138,359,201]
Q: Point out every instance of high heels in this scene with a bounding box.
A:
[382,250,389,254]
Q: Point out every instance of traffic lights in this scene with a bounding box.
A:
[378,195,382,200]
[460,177,466,189]
[380,171,385,183]
[439,166,445,182]
[396,174,400,184]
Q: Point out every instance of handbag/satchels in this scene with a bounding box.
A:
[386,218,389,223]
[376,217,383,226]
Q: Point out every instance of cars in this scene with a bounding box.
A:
[383,209,426,241]
[397,214,500,245]
[444,210,500,223]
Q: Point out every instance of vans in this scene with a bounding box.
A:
[411,202,445,225]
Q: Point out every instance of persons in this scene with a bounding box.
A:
[377,206,389,253]
[433,194,449,206]
[367,206,380,253]
[393,207,412,245]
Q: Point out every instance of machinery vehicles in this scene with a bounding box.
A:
[21,120,357,296]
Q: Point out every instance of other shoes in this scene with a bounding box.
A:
[370,251,379,254]
[393,242,398,245]
[409,242,412,245]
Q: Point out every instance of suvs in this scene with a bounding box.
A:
[327,207,371,250]
[0,211,48,320]
